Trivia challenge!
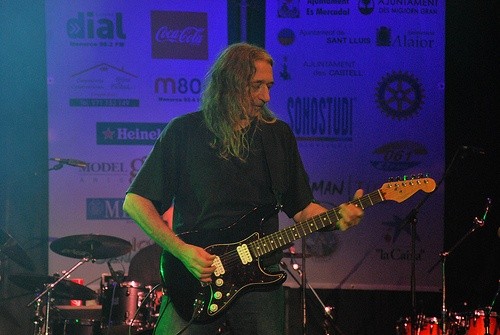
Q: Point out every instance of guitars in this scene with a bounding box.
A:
[160,173,436,324]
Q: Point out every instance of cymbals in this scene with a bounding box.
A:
[50,233,133,259]
[9,273,98,298]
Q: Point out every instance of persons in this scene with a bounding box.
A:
[128,203,173,325]
[123,44,364,335]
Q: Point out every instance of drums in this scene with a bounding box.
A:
[405,316,454,335]
[463,309,500,335]
[103,279,155,335]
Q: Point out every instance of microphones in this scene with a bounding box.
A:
[49,157,88,167]
[107,262,119,281]
[462,145,489,155]
